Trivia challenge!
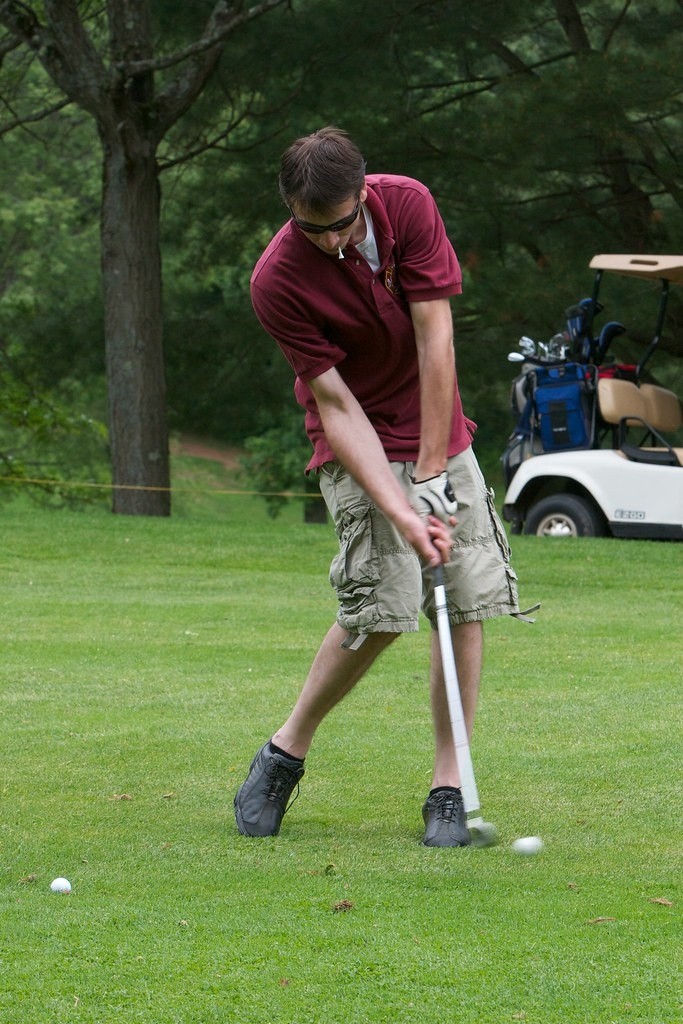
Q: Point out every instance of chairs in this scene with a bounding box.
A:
[596,377,683,466]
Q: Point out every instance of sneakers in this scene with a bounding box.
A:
[234,736,305,838]
[422,786,471,848]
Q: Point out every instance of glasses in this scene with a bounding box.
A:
[289,194,360,234]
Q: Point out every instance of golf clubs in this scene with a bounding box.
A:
[507,332,570,363]
[434,563,498,848]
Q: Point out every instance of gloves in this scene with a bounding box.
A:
[406,470,458,540]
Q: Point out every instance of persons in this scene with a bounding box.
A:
[231,129,541,849]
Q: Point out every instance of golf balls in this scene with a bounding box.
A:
[512,837,543,856]
[50,878,72,892]
